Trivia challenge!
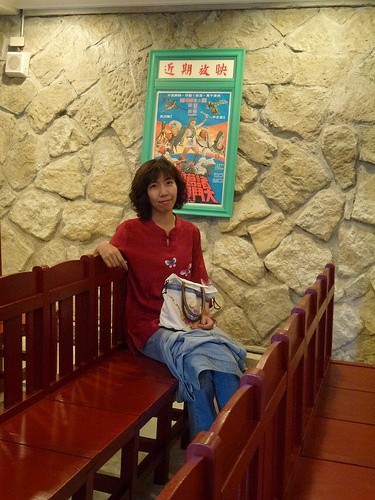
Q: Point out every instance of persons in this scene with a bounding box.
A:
[93,158,241,445]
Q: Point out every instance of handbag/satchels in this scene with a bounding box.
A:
[158,273,217,333]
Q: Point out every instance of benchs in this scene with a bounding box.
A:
[0,250,191,499]
[156,263,375,499]
